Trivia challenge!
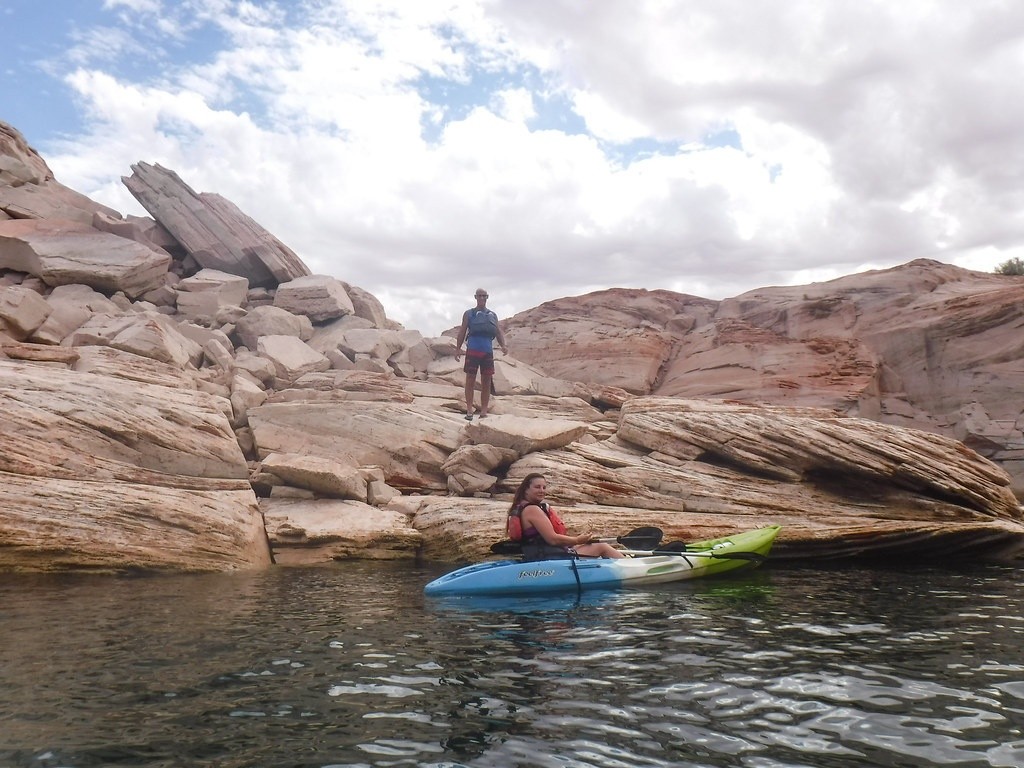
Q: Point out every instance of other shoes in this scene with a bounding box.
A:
[465,415,473,420]
[479,415,486,419]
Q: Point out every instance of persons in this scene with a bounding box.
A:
[505,473,625,563]
[454,288,508,421]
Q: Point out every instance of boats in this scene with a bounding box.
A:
[423,524,783,599]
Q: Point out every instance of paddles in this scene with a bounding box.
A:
[489,525,772,563]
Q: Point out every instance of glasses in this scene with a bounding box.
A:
[476,295,488,299]
